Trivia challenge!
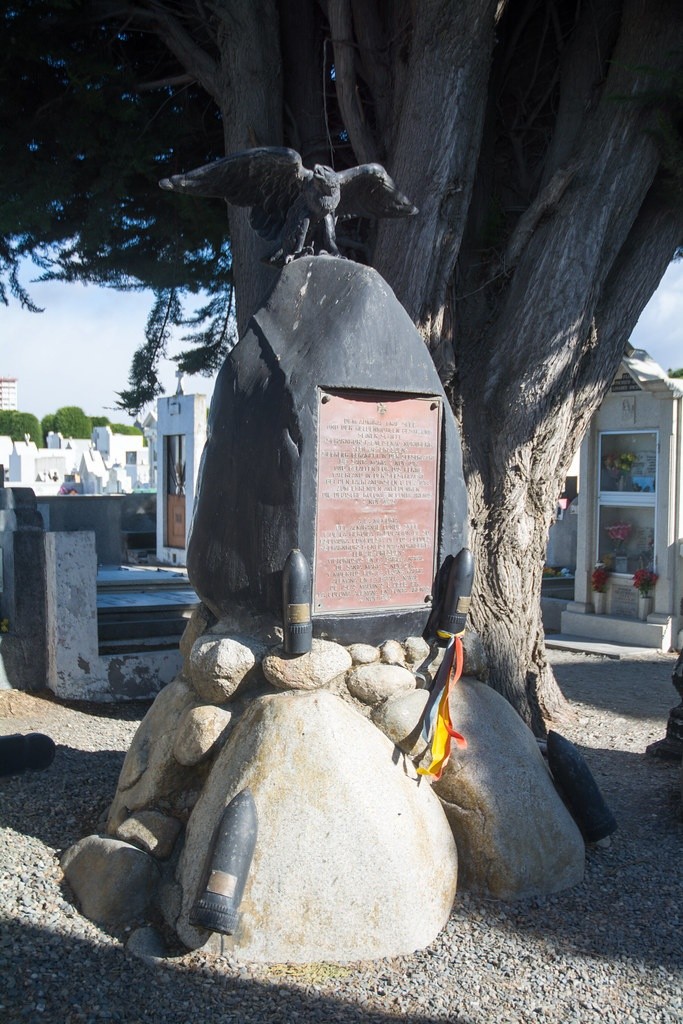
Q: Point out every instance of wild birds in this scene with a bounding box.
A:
[158,146,421,268]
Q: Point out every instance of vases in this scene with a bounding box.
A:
[615,557,629,573]
[594,591,607,615]
[616,475,627,490]
[638,595,651,621]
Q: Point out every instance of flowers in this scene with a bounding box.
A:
[604,523,633,555]
[632,569,658,597]
[601,453,638,473]
[591,566,609,592]
